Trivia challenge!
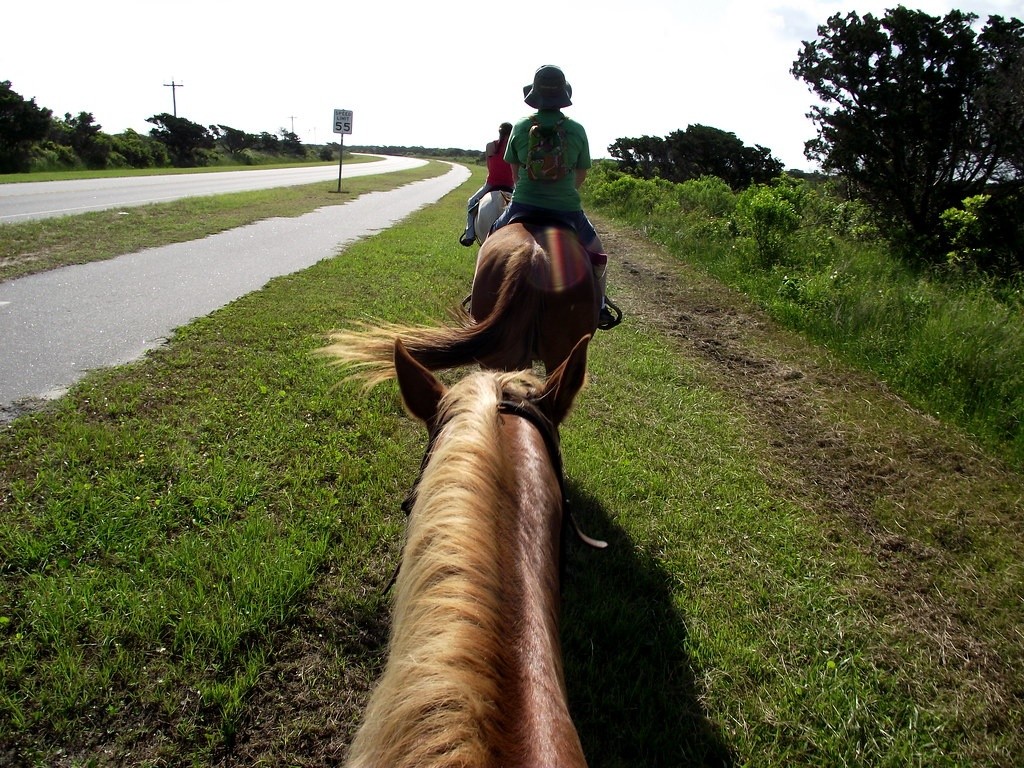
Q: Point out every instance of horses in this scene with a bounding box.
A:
[310,222,603,430]
[474,189,513,246]
[344,335,591,767]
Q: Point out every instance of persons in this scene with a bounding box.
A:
[460,123,514,244]
[483,64,616,326]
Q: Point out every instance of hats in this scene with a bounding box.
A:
[523,64,572,110]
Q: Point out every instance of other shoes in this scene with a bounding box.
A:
[462,237,475,246]
[598,305,615,327]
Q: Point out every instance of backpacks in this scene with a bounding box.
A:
[520,115,577,183]
